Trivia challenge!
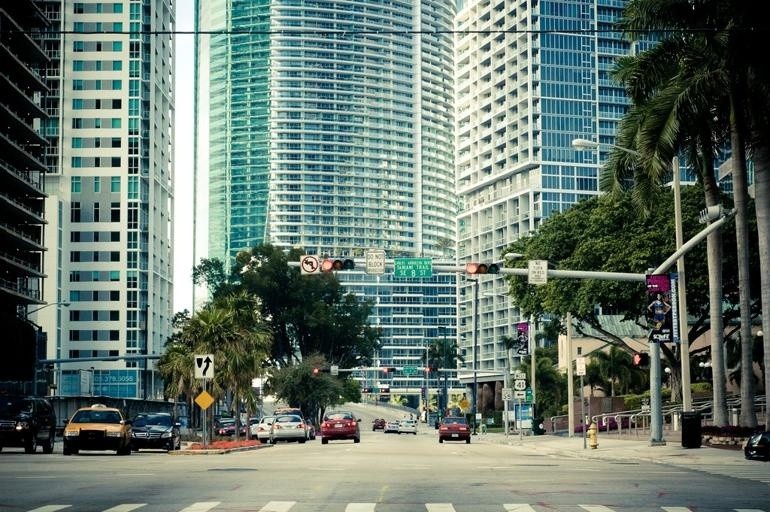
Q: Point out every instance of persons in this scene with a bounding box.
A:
[648,293,671,327]
[519,332,528,350]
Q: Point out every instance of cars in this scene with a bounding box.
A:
[371,418,418,435]
[216,408,316,444]
[0,397,181,455]
[435,416,471,444]
[320,410,362,444]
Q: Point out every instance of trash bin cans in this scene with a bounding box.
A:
[531,417,544,435]
[679,410,702,449]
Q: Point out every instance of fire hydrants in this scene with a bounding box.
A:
[585,419,599,449]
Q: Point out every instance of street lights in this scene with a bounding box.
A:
[571,139,692,412]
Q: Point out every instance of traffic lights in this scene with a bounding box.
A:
[384,367,396,373]
[321,258,355,273]
[466,262,500,275]
[424,367,438,372]
[312,368,319,374]
